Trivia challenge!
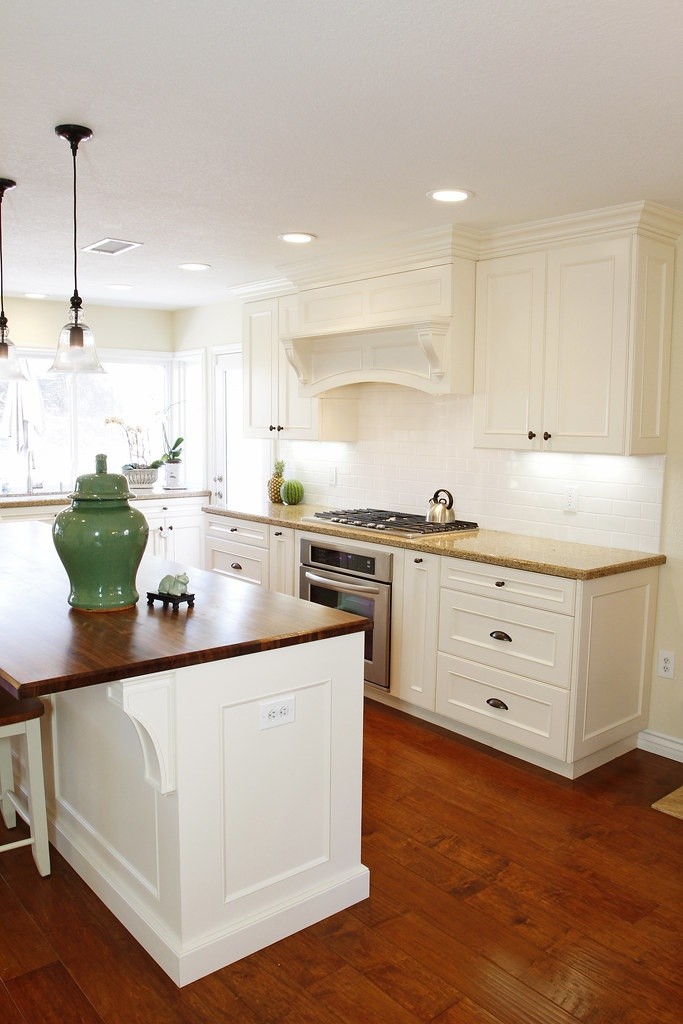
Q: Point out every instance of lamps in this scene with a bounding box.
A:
[48,124,110,385]
[0,179,17,365]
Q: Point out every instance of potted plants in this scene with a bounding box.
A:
[152,424,185,490]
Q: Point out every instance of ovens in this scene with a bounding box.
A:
[295,537,393,693]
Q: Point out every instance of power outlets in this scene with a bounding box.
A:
[254,703,293,728]
[656,650,676,679]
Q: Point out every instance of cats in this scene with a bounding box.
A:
[157,572,190,597]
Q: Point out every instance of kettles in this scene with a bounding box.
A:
[426,489,456,523]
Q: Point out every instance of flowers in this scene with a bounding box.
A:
[104,417,153,469]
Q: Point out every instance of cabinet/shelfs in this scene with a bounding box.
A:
[239,244,675,455]
[1,524,661,780]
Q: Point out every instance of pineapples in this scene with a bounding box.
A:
[267,459,287,503]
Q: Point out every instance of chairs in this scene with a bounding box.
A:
[0,689,51,878]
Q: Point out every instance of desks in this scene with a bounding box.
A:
[0,526,370,988]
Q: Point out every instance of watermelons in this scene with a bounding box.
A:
[279,479,304,504]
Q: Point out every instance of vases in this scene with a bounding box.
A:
[124,467,158,489]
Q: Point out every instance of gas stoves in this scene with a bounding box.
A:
[302,508,480,538]
